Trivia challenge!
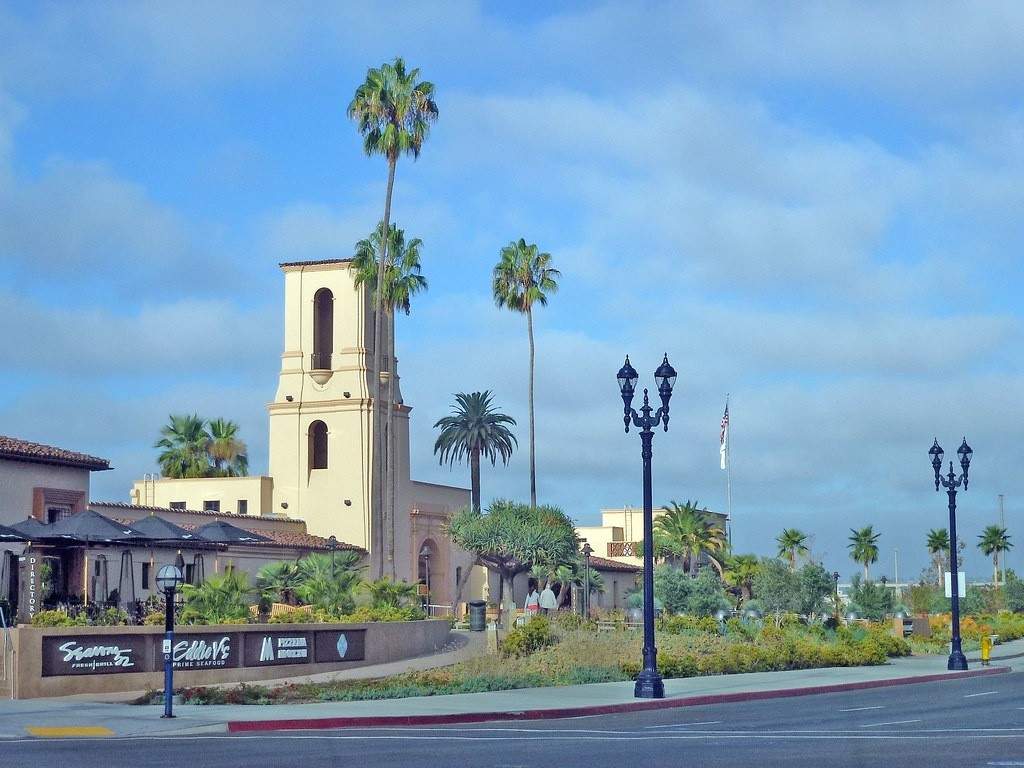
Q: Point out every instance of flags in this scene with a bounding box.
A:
[720,400,729,469]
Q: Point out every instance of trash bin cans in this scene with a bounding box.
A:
[470,599,486,631]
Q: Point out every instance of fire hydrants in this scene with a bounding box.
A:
[979,637,993,665]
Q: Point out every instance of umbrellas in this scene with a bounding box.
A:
[0,506,275,607]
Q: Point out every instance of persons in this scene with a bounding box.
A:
[539,583,558,616]
[525,586,539,615]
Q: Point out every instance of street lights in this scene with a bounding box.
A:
[326,536,342,576]
[616,351,677,699]
[155,564,185,719]
[928,436,973,672]
[420,546,434,619]
[580,543,595,618]
